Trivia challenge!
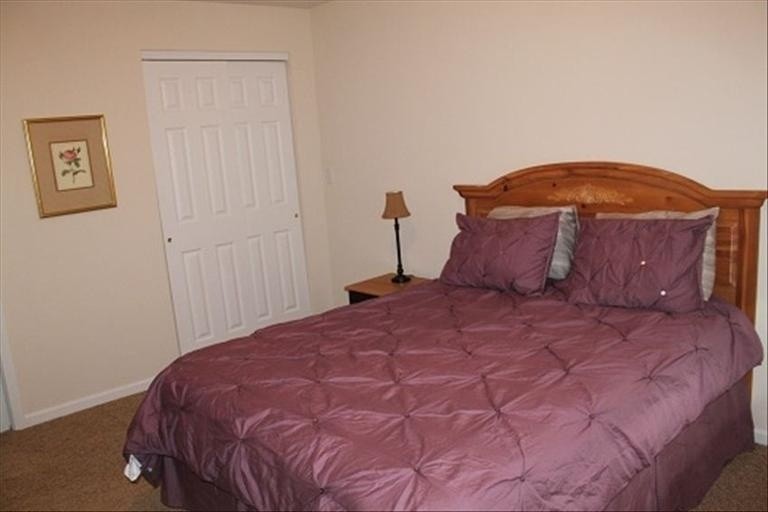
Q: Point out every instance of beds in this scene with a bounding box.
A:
[152,164,768,510]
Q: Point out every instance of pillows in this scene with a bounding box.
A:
[438,207,720,318]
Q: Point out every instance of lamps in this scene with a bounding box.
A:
[379,189,411,285]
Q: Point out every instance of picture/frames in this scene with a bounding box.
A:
[20,114,118,218]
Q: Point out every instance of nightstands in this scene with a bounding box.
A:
[344,270,434,305]
